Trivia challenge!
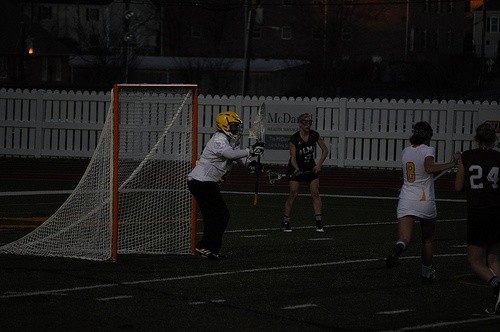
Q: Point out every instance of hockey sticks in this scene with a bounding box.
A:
[268,170,314,185]
[248,102,266,207]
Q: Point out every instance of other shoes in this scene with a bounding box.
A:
[422,269,441,280]
[386,241,406,268]
[486,281,500,314]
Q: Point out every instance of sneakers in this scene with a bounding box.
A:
[280,221,292,232]
[315,221,324,232]
[195,248,221,260]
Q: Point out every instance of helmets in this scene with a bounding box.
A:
[215,111,243,142]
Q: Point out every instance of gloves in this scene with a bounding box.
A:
[248,160,259,174]
[246,142,264,157]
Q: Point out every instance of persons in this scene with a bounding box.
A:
[279,114,328,232]
[454,124,500,313]
[187,111,265,261]
[384,122,460,280]
